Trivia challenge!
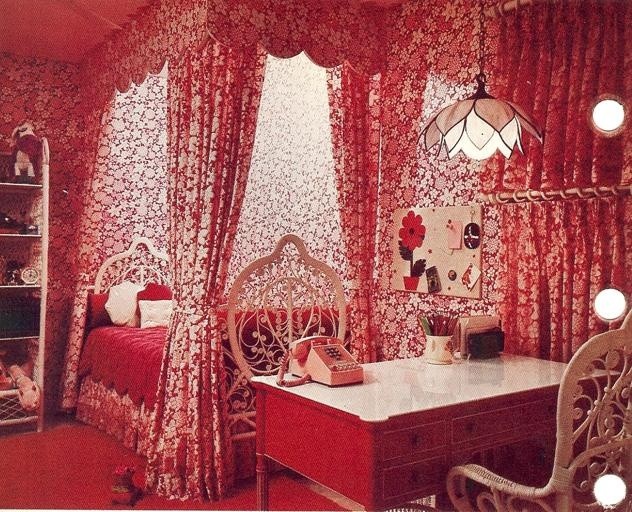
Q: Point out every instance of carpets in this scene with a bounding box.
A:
[0,424,349,510]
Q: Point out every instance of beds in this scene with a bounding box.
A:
[74,238,350,504]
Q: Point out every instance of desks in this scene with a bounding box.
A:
[250,349,623,511]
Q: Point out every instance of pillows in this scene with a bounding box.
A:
[86,282,174,332]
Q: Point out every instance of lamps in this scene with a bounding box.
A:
[415,0,543,163]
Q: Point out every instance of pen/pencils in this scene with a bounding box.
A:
[419,308,460,335]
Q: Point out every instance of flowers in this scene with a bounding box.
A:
[398,211,426,278]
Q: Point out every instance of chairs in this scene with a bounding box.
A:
[446,309,632,512]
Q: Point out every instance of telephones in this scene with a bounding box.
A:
[289,336,363,386]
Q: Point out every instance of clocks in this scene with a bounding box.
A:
[21,267,39,284]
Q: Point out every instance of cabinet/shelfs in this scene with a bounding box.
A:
[0,138,49,432]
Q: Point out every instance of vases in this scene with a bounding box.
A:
[403,275,419,290]
